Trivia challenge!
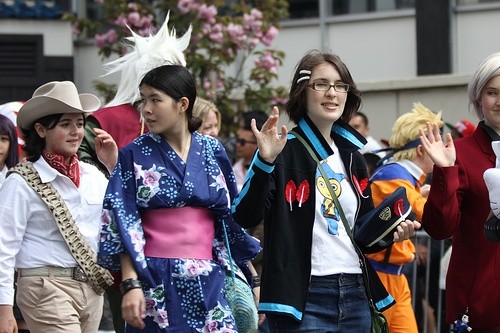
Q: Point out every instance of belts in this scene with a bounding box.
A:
[19,265,87,280]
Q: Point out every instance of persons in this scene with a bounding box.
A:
[348,110,388,166]
[229,48,421,331]
[353,102,445,333]
[0,80,118,333]
[191,96,221,140]
[1,114,20,190]
[361,152,385,181]
[418,51,500,332]
[238,107,270,141]
[97,64,266,333]
[231,127,266,194]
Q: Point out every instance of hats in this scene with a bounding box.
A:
[17,80,101,131]
[445,121,475,137]
[354,186,416,253]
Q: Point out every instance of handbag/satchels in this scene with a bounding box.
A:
[369,306,388,333]
[224,276,258,333]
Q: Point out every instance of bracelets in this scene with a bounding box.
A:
[119,277,142,296]
[251,275,261,288]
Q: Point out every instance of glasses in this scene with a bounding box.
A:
[307,82,351,93]
[236,138,256,147]
